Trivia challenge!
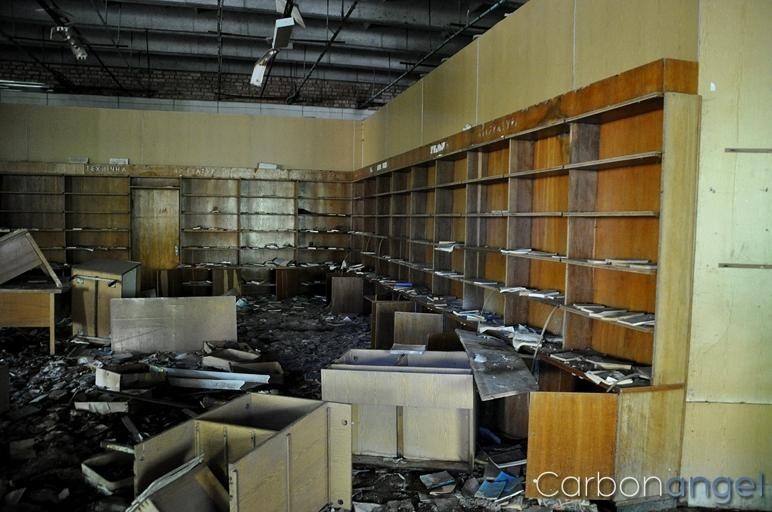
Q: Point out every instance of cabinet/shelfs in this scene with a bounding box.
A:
[134,393,353,512]
[0,161,352,294]
[320,348,476,475]
[68,258,140,348]
[357,56,702,502]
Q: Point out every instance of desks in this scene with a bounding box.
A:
[0,290,61,355]
[0,229,62,288]
